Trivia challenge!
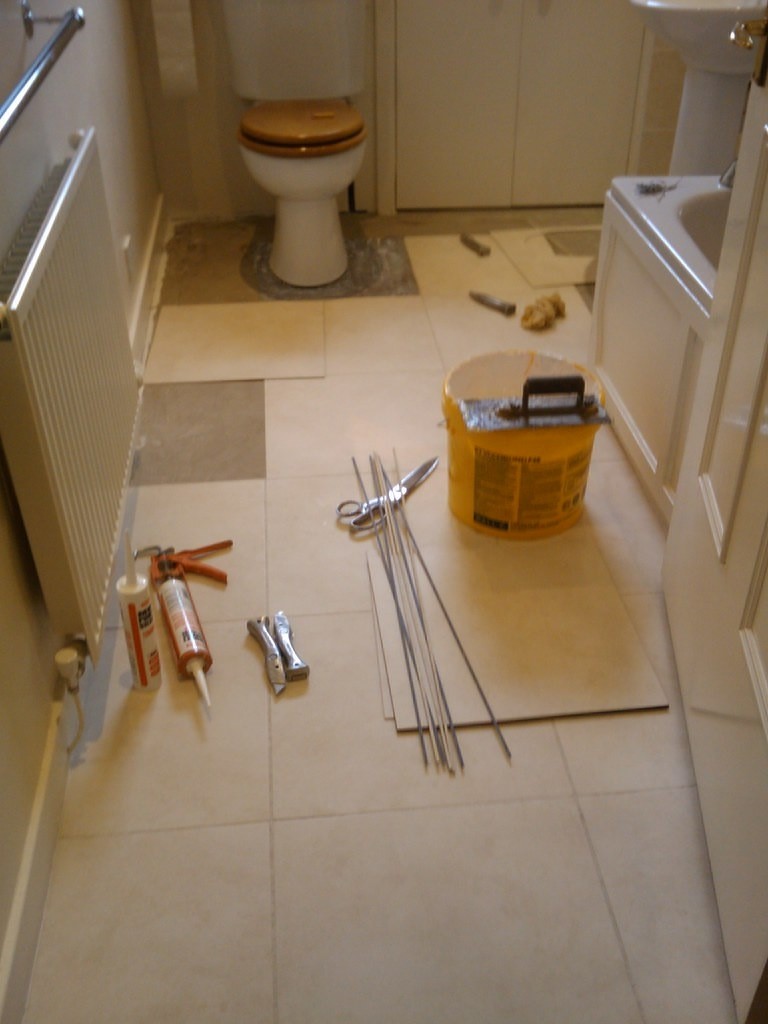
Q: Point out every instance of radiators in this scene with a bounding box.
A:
[0,126,141,669]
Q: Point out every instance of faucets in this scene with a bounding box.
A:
[718,160,738,187]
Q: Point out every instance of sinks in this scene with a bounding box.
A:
[675,188,736,273]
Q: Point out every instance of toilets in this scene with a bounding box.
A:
[221,0,370,288]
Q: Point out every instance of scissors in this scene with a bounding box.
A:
[336,457,440,530]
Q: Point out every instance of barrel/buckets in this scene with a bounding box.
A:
[443,351,605,540]
